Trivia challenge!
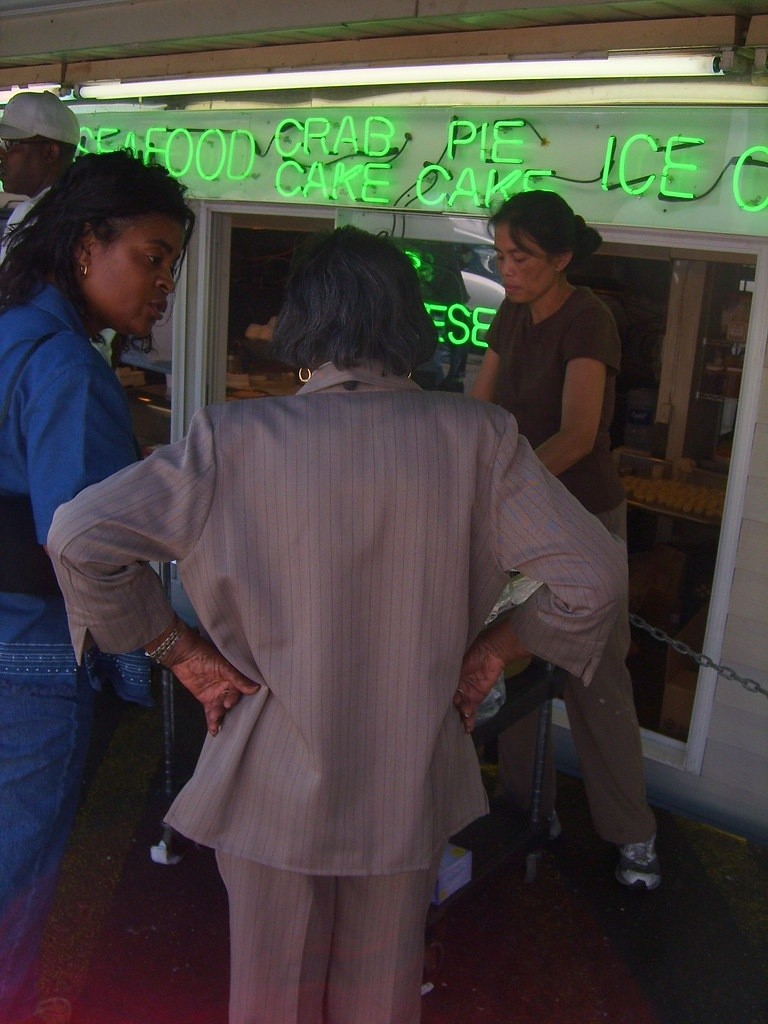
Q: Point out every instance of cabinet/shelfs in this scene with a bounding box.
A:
[147,557,565,940]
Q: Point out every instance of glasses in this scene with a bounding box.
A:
[0,138,50,153]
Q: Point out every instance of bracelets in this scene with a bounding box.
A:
[145,614,188,664]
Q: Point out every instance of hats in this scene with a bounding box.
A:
[0,90,81,145]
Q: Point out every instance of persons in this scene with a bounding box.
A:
[468,190,666,892]
[0,151,196,1023]
[0,91,79,263]
[46,221,628,1024]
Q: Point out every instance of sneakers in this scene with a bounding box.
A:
[523,807,563,841]
[615,828,662,890]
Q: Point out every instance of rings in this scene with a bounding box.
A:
[457,687,464,693]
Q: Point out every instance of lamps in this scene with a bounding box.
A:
[73,49,747,101]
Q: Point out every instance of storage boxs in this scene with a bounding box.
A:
[432,843,473,907]
